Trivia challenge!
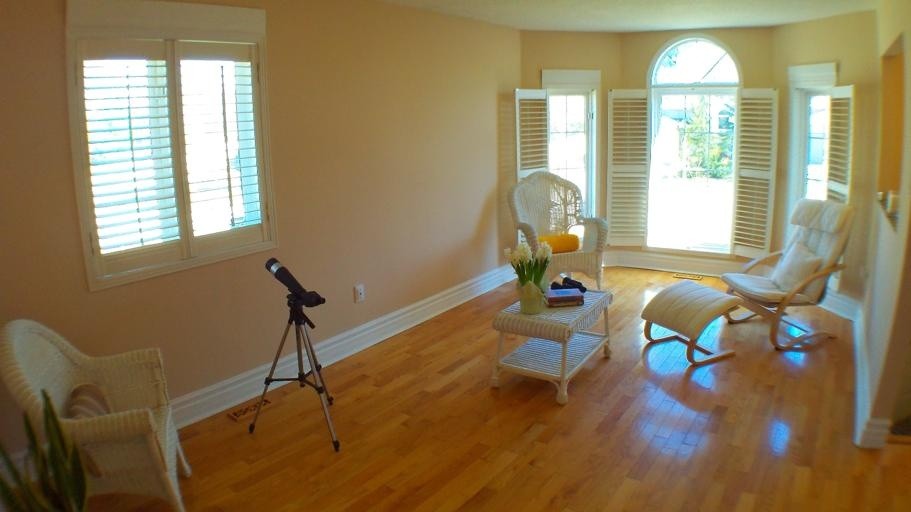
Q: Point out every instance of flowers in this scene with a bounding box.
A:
[505,242,552,284]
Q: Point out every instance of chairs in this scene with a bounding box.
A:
[2,318,192,511]
[724,197,856,351]
[508,169,609,289]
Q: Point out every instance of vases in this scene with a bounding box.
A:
[515,278,543,315]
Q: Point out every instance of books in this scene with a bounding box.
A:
[548,301,584,306]
[545,288,583,302]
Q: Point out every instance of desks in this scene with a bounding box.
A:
[489,289,615,406]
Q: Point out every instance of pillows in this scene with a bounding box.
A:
[66,382,115,422]
[540,232,581,254]
[771,243,821,294]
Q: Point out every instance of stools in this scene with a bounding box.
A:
[640,280,742,367]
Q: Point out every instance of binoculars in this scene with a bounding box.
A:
[265,258,325,308]
